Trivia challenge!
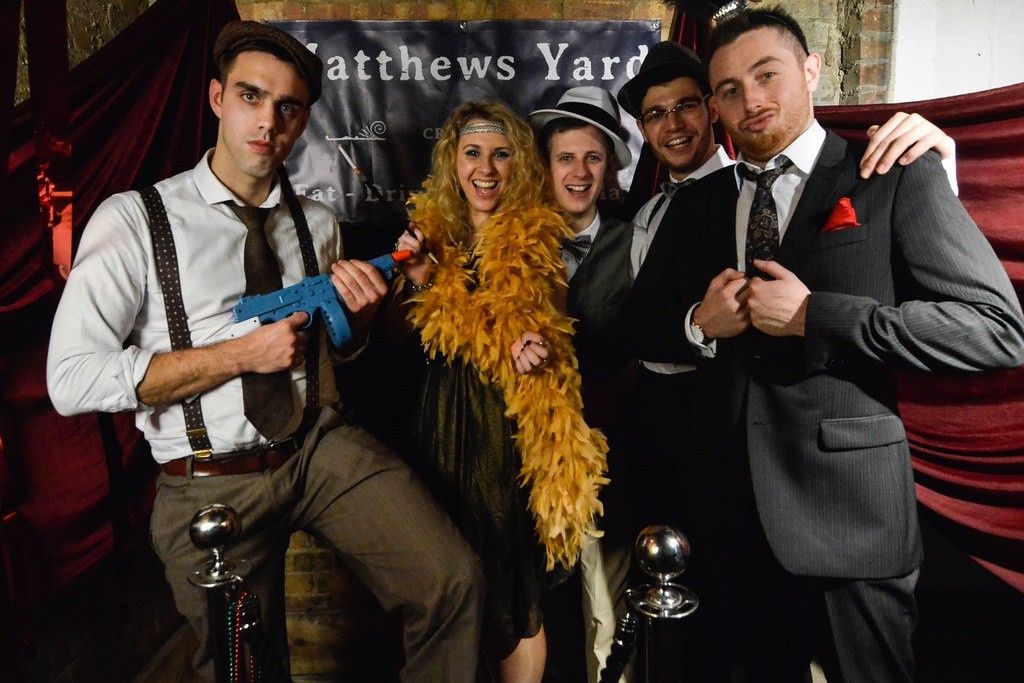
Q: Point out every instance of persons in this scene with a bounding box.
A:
[620,4,1024,683]
[527,87,651,683]
[616,41,958,683]
[47,19,492,683]
[396,97,548,683]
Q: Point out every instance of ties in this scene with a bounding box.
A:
[736,161,793,276]
[225,205,295,440]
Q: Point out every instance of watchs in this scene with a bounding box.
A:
[690,303,715,346]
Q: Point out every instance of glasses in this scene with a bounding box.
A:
[639,93,710,123]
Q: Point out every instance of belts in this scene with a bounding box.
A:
[162,437,300,479]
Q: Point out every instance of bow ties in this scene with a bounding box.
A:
[658,178,696,199]
[559,235,590,266]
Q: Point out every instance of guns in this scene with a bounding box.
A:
[231,249,411,348]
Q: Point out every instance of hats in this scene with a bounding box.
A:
[616,41,713,117]
[528,84,632,172]
